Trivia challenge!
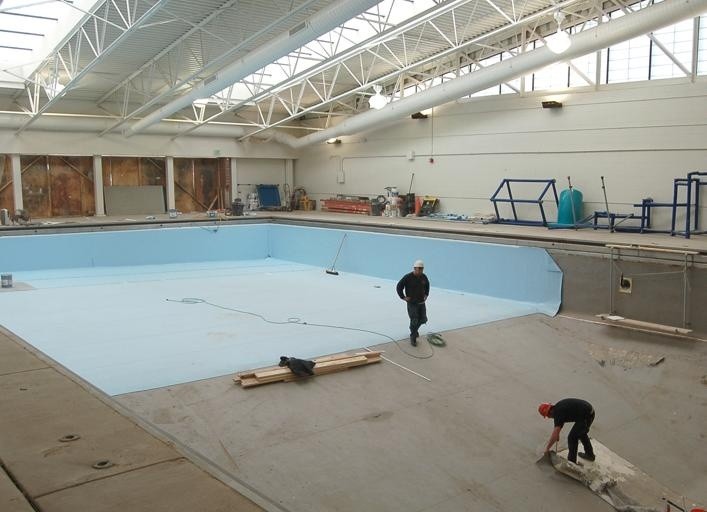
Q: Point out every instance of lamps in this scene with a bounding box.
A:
[326,136,342,145]
[368,82,388,111]
[410,110,430,119]
[542,9,571,58]
[542,99,563,108]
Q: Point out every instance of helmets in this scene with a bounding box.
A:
[414,261,424,268]
[539,403,552,418]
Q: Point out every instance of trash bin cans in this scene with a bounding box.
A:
[1,274,12,287]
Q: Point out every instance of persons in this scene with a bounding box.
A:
[395,258,430,349]
[535,395,595,466]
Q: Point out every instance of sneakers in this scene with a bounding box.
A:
[411,332,419,346]
[578,452,595,461]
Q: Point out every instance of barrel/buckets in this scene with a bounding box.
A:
[0,272,13,287]
[169,209,177,219]
